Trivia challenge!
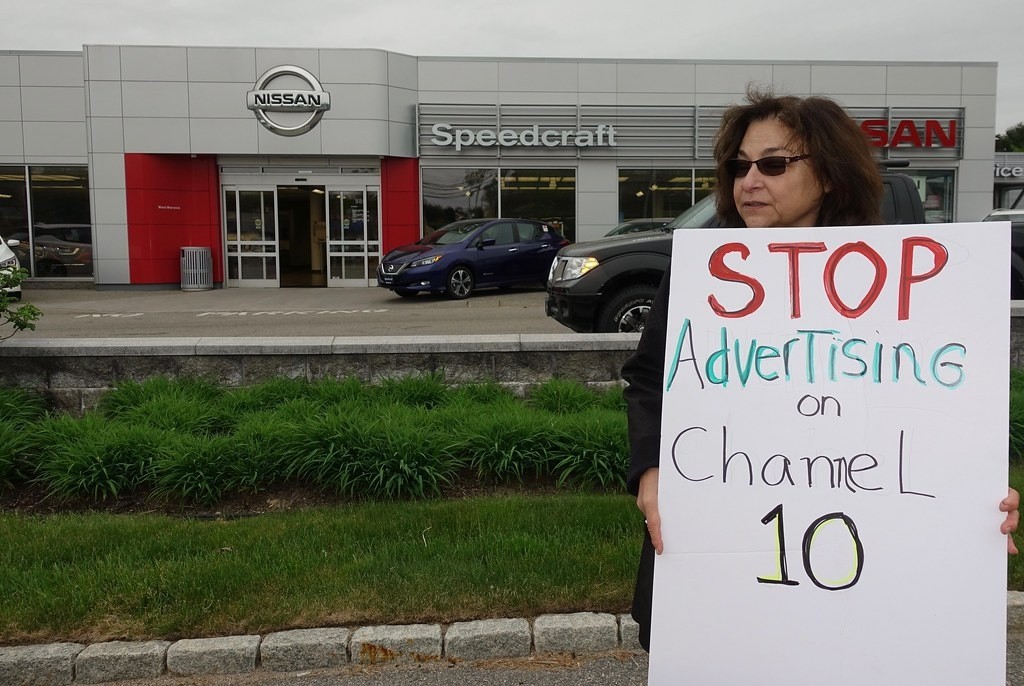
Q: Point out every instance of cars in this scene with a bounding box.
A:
[603,218,676,238]
[227,212,264,255]
[376,218,571,300]
[0,234,22,302]
[32,221,92,276]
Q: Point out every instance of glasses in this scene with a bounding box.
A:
[727,154,811,178]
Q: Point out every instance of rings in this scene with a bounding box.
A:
[645,520,647,524]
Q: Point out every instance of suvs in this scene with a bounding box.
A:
[545,149,925,333]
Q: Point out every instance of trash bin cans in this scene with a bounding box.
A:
[179,246,213,290]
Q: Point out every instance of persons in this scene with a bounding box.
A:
[621,98,1019,651]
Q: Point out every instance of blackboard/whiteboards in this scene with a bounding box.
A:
[645,220,1013,686]
